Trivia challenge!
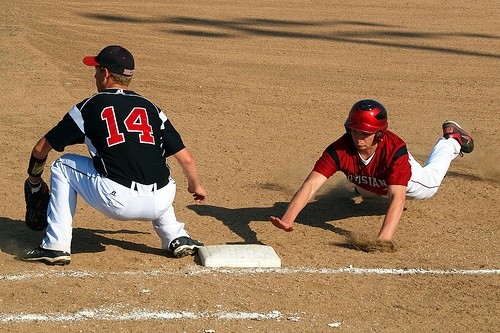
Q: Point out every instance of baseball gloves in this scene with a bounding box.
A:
[24,177,50,232]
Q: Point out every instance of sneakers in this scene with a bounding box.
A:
[22,247,71,266]
[170,237,205,258]
[443,120,475,158]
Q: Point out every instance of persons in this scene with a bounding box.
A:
[268,100,475,242]
[19,45,206,265]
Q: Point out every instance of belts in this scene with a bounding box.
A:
[99,169,169,191]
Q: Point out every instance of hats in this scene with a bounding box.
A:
[83,45,135,77]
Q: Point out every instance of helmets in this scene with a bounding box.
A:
[345,100,389,142]
